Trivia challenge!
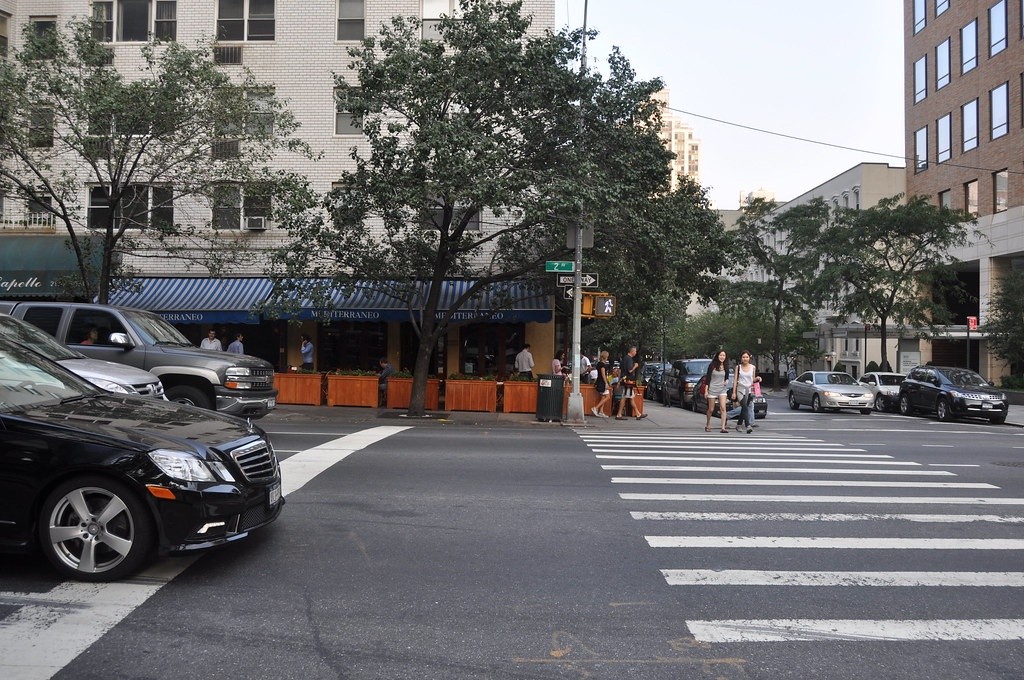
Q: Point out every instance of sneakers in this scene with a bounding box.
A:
[590,406,599,416]
[597,412,609,418]
[736,424,743,432]
[746,425,753,433]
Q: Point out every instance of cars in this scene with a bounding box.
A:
[787,371,874,414]
[641,358,765,421]
[856,373,905,413]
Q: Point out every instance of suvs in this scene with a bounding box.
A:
[900,365,1010,424]
[0,301,283,583]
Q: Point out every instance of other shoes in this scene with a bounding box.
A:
[636,413,647,420]
[615,415,628,420]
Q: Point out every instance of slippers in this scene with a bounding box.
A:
[719,428,728,433]
[705,426,712,432]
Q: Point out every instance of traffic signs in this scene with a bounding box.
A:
[557,272,599,299]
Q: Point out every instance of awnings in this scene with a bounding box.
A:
[92,278,287,324]
[261,277,553,324]
[0,235,116,299]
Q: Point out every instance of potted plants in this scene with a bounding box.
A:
[326,369,381,408]
[384,370,439,410]
[501,374,615,414]
[272,367,325,407]
[630,378,648,418]
[444,372,501,412]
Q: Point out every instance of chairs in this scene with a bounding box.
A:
[322,369,332,394]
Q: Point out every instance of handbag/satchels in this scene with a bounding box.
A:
[727,388,738,402]
[700,364,715,394]
[751,380,762,398]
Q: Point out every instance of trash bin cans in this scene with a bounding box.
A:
[537,373,567,422]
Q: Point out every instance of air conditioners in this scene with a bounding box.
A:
[241,215,267,230]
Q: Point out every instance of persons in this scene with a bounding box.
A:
[226,333,244,354]
[515,344,534,379]
[200,328,223,350]
[717,400,759,427]
[704,350,729,433]
[80,323,98,344]
[591,351,610,418]
[580,349,591,384]
[787,365,796,382]
[300,334,314,371]
[379,357,395,389]
[731,349,762,433]
[552,350,566,375]
[615,346,648,420]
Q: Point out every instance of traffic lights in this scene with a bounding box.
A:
[595,295,615,316]
